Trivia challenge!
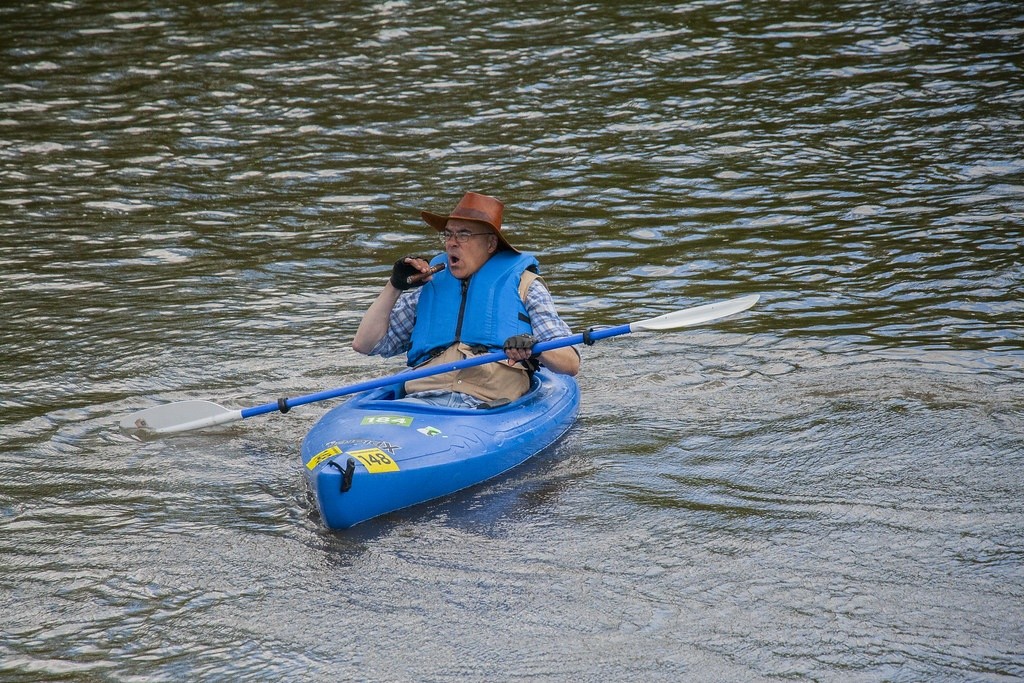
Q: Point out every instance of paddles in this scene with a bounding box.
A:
[116,293,763,433]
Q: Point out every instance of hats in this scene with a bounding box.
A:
[421,191,522,255]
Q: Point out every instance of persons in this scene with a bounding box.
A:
[352,192,581,409]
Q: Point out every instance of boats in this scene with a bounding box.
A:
[300,366,582,534]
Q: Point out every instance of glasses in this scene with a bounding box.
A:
[438,228,496,243]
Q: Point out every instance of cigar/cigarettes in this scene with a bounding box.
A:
[407,263,445,284]
